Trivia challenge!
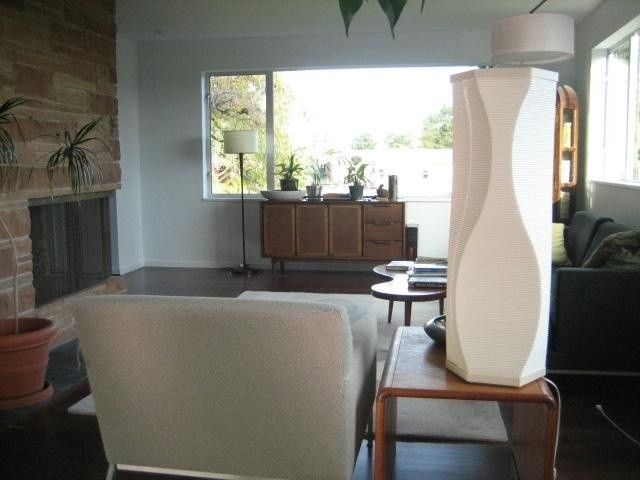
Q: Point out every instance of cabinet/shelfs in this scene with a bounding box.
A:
[362,202,405,262]
[258,202,295,259]
[295,202,363,261]
[553,84,580,205]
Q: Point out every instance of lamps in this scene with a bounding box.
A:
[492,13,575,66]
[223,130,259,276]
[446,67,560,390]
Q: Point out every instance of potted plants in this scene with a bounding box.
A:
[271,152,303,191]
[344,159,368,200]
[0,96,117,411]
[304,156,331,198]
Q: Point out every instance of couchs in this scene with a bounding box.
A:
[545,211,639,398]
[71,296,376,479]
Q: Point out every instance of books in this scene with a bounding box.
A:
[386,257,447,288]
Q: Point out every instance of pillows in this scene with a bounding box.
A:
[601,247,640,272]
[582,229,640,269]
[552,222,573,266]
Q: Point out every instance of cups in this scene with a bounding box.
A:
[389,175,397,202]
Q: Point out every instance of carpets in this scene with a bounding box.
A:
[233,291,509,442]
